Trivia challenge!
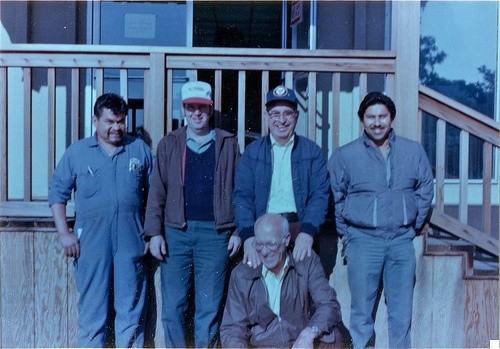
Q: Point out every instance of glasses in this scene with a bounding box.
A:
[269,110,296,119]
[185,104,212,111]
[252,233,286,251]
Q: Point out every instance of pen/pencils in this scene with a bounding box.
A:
[86,165,95,177]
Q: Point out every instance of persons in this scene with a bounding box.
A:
[218,212,353,349]
[143,79,243,349]
[46,91,155,349]
[230,84,331,271]
[325,90,435,349]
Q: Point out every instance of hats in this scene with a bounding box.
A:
[181,81,213,105]
[358,91,396,120]
[265,86,297,105]
